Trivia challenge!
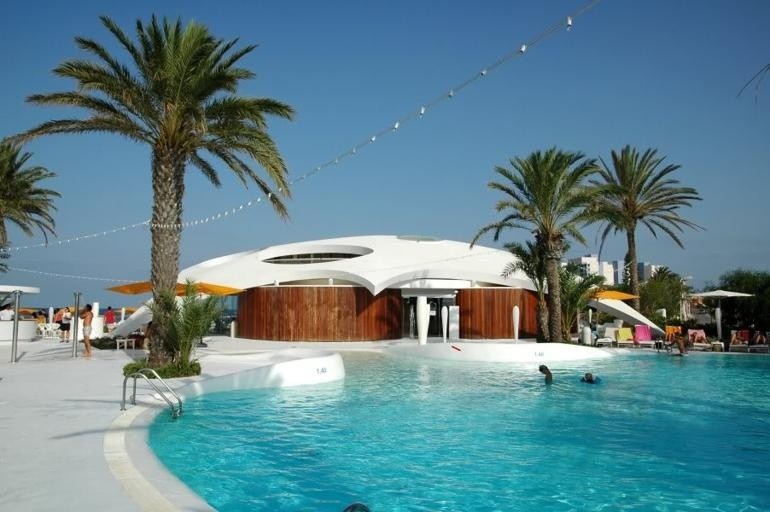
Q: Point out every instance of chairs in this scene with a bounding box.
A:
[38,322,62,341]
[594,326,770,353]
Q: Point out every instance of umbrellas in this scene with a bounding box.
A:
[688,290,754,341]
[590,290,640,331]
[107,281,248,343]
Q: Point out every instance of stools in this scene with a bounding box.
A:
[115,337,136,351]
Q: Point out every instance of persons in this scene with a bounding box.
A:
[0,304,116,357]
[539,365,552,384]
[581,373,594,384]
[654,331,766,353]
